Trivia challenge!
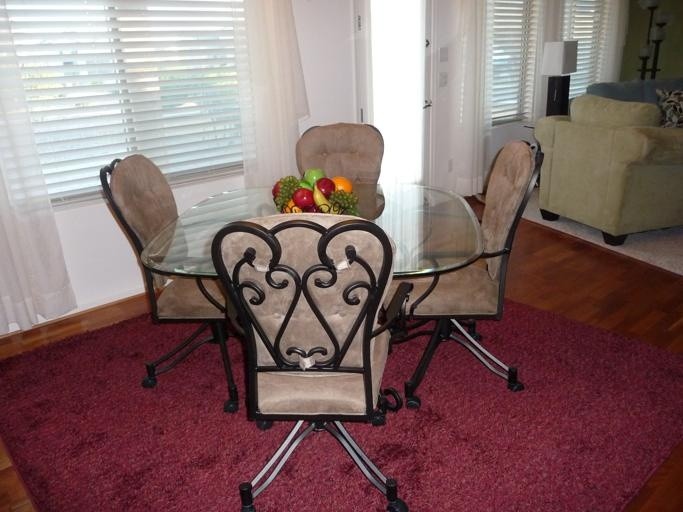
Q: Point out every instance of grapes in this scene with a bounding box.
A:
[275,176,300,210]
[330,189,360,216]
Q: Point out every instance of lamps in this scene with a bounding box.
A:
[636,0,666,80]
[540,40,577,115]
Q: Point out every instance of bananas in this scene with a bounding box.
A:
[312,184,341,214]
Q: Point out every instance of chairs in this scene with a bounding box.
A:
[382,135,543,409]
[210,218,408,511]
[99,153,237,414]
[530,94,682,245]
[295,122,382,185]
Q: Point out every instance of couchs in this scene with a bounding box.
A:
[586,77,681,102]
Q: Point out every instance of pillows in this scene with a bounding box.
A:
[655,89,682,127]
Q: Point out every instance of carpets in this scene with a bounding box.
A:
[0,297,681,508]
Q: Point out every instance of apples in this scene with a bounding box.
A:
[304,167,326,188]
[304,205,321,213]
[293,188,314,210]
[299,182,314,192]
[272,181,283,197]
[317,178,335,199]
